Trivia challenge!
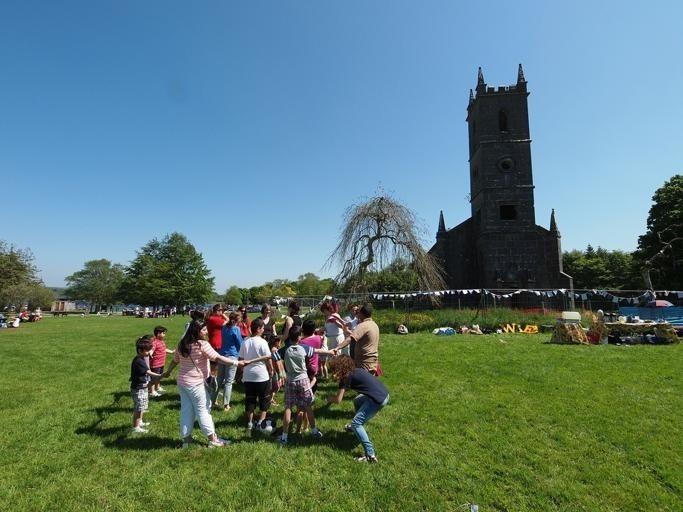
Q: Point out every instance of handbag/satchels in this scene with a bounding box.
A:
[205,375,219,402]
[376,365,382,376]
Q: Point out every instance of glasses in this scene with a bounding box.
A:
[240,310,246,312]
[217,309,224,311]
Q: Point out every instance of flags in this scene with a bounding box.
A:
[644,292,650,298]
[534,291,540,297]
[434,291,440,296]
[462,290,468,294]
[581,293,587,300]
[601,291,608,297]
[546,291,553,297]
[632,297,639,303]
[612,296,618,303]
[377,295,382,300]
[540,291,546,296]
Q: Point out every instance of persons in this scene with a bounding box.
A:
[127,297,384,449]
[324,353,390,462]
[10,315,20,327]
[21,309,29,322]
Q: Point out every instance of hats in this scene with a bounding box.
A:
[327,313,341,320]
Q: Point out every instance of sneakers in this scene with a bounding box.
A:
[345,425,352,429]
[276,435,288,443]
[311,430,322,438]
[208,438,231,448]
[354,455,378,463]
[248,420,262,430]
[149,387,167,397]
[132,420,150,433]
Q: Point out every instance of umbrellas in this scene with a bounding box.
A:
[644,299,675,318]
